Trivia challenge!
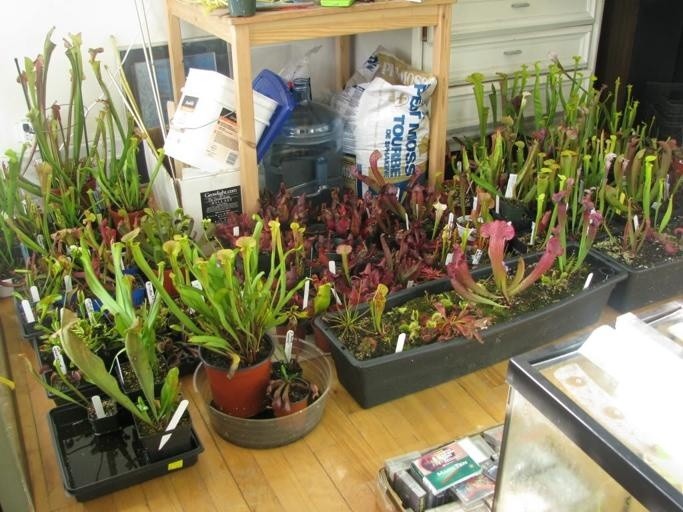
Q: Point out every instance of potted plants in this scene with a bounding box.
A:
[449,55,682,312]
[122,214,331,449]
[215,149,633,409]
[1,39,204,501]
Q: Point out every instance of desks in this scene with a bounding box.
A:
[165,1,459,220]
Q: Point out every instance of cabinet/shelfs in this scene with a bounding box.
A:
[490,300,683,511]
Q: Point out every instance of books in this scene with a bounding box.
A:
[383,420,594,512]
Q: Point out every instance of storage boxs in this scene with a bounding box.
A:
[373,419,591,512]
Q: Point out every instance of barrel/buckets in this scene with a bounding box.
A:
[258,77,346,235]
